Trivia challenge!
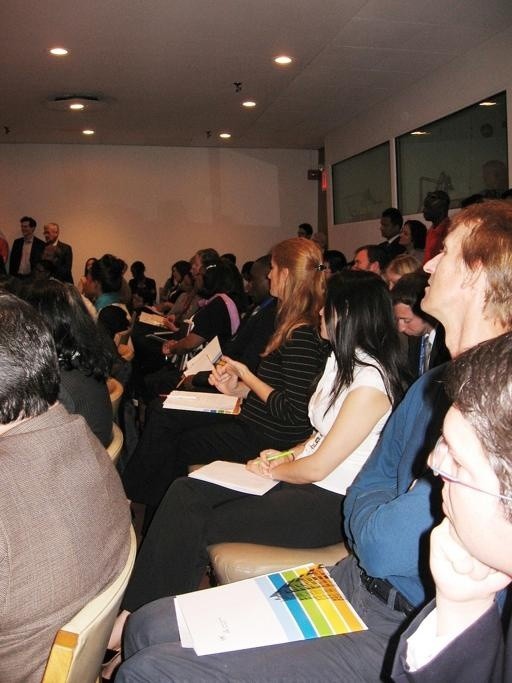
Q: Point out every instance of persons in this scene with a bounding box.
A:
[104,269,417,680]
[0,218,158,337]
[460,160,509,205]
[114,196,510,681]
[0,285,134,683]
[384,331,510,680]
[124,190,450,529]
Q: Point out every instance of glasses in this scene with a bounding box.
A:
[426,433,512,506]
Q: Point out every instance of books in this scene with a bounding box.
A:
[173,556,371,659]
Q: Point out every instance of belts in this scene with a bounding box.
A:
[360,566,423,620]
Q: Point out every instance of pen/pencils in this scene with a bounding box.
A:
[154,331,174,335]
[176,377,186,389]
[159,394,197,399]
[254,450,295,465]
